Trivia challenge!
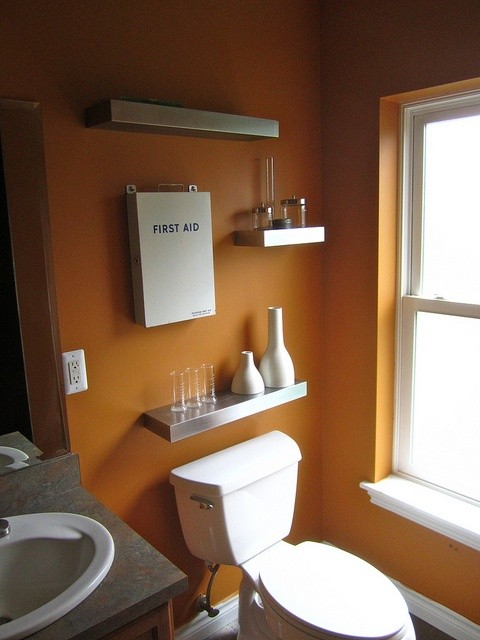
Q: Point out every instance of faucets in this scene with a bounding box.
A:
[0,518,10,538]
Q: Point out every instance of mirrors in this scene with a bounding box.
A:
[0,99,71,477]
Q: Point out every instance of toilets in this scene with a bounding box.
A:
[171,428,417,640]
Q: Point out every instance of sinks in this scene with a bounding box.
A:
[0,511,115,640]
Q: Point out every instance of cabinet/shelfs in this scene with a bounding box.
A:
[85,98,327,443]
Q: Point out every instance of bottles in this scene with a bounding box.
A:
[281,195,306,228]
[201,364,217,403]
[185,367,202,408]
[170,370,187,411]
[252,202,273,230]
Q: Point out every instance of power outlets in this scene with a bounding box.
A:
[62,349,89,397]
